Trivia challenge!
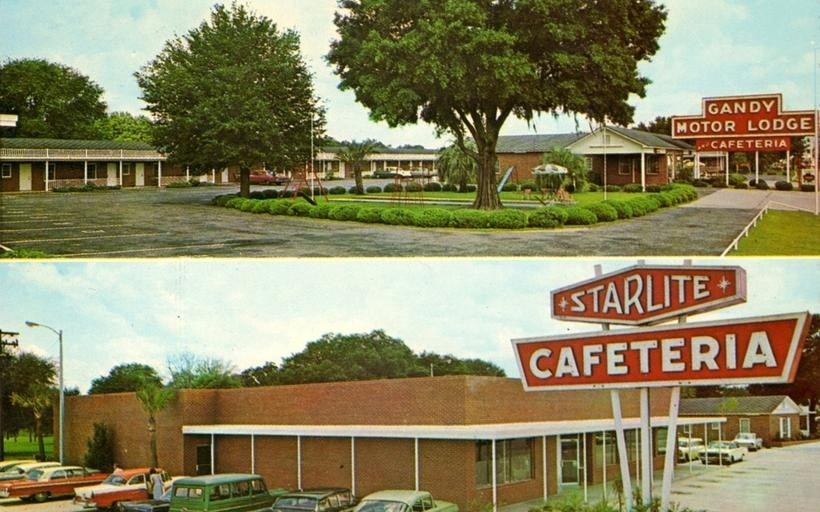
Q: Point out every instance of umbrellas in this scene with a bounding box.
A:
[529,164,568,174]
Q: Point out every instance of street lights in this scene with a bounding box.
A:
[25,319,66,465]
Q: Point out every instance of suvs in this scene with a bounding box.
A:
[167,470,291,512]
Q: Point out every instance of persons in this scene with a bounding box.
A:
[113,462,123,484]
[150,467,165,499]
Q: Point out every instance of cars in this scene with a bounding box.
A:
[115,485,198,512]
[0,456,113,503]
[374,165,435,180]
[270,486,360,511]
[248,169,292,187]
[697,439,749,466]
[678,435,705,462]
[353,488,461,512]
[732,431,764,451]
[73,464,190,512]
